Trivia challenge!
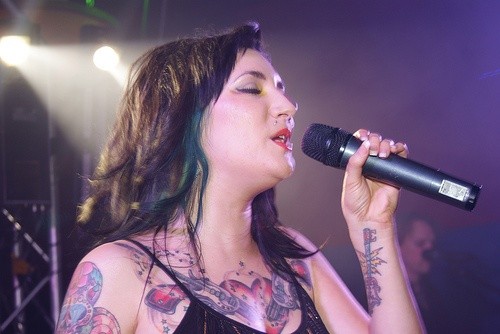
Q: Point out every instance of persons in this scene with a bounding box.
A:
[55,22,428,334]
[397,213,500,333]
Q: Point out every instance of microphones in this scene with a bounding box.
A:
[301,123,480,211]
